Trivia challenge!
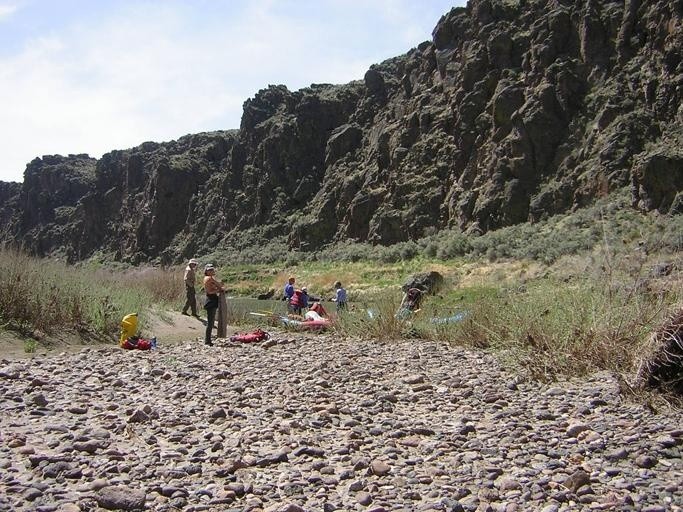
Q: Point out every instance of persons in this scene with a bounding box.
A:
[285,277,295,313]
[202,263,225,346]
[330,281,347,313]
[290,287,309,318]
[181,258,200,317]
[400,283,422,310]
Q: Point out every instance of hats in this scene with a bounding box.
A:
[205,264,214,270]
[302,287,307,293]
[187,259,198,265]
